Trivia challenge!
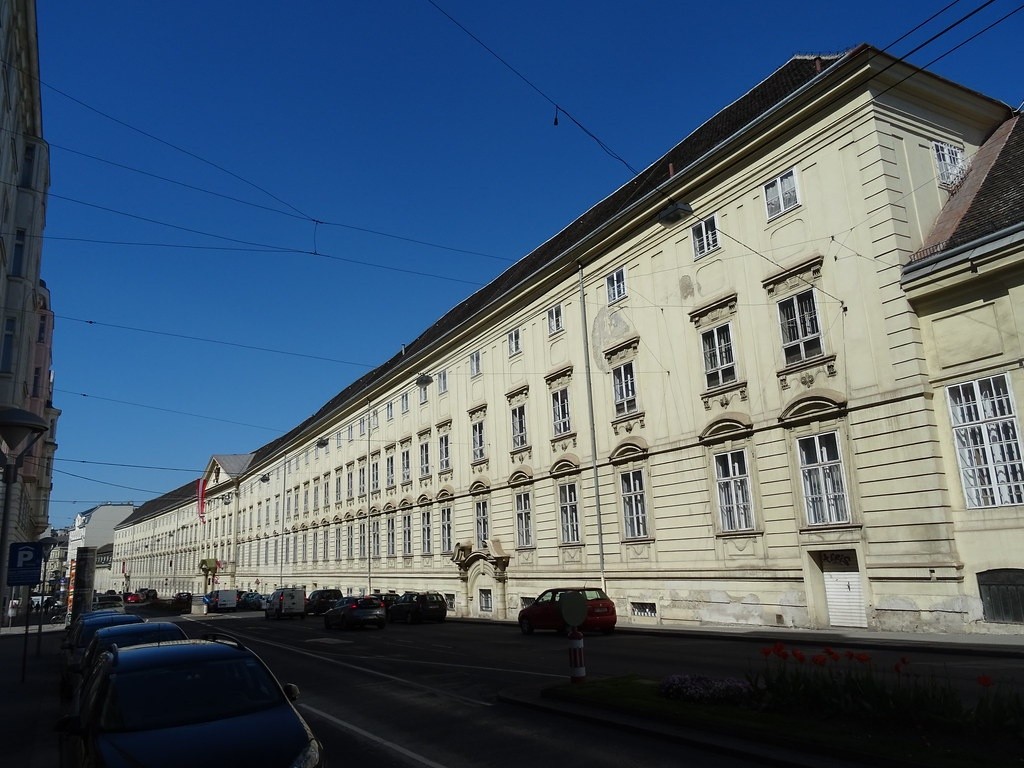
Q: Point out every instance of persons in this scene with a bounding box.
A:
[34,601,40,615]
[43,597,57,615]
[28,598,34,612]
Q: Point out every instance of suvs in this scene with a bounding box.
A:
[205,586,401,629]
[79,632,325,768]
[385,591,449,625]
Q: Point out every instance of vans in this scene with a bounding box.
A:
[92,593,126,614]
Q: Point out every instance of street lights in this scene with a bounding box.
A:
[34,536,58,665]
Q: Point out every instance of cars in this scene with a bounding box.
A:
[106,588,194,617]
[517,588,618,634]
[61,612,188,666]
